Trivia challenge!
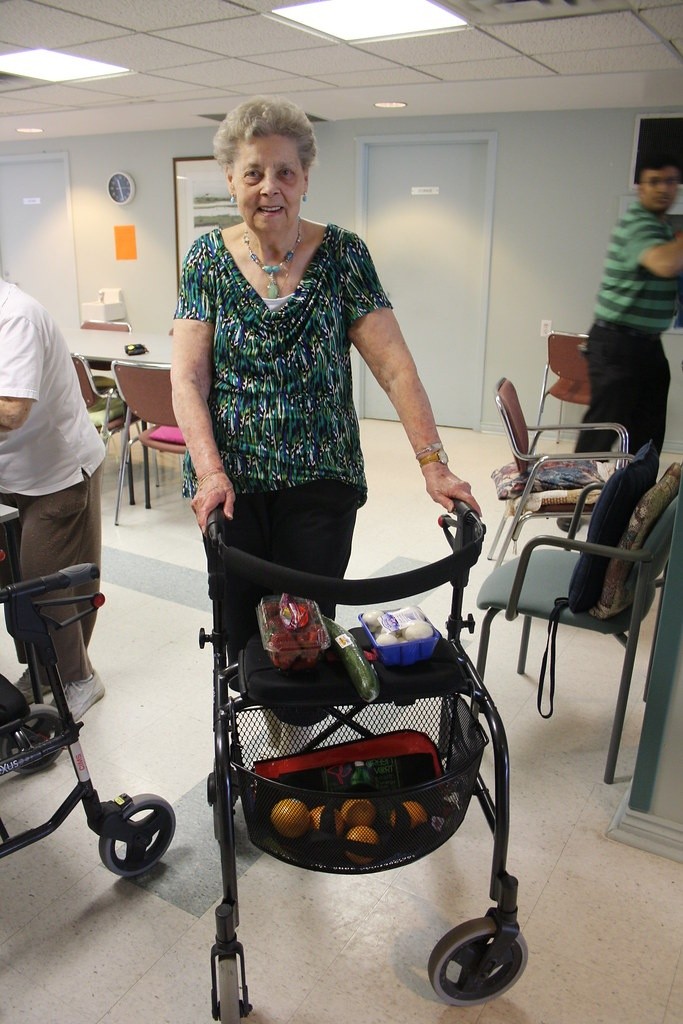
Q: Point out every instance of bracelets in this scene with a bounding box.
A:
[415,442,442,458]
[195,473,219,485]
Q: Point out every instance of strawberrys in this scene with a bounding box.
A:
[265,603,324,672]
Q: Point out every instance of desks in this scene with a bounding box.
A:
[71,328,173,364]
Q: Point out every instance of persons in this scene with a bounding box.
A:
[0,275,107,728]
[170,94,484,752]
[554,153,683,531]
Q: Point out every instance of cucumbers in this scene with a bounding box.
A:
[321,615,380,702]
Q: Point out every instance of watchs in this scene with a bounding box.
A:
[418,448,449,468]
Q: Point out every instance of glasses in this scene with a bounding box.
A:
[639,176,677,186]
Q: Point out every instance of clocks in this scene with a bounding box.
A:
[107,172,135,206]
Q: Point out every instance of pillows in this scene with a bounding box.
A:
[567,440,659,613]
[492,457,605,499]
[589,461,680,620]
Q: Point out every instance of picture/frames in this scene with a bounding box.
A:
[173,156,244,295]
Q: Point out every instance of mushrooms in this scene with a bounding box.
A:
[360,607,434,646]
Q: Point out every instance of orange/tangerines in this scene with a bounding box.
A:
[269,797,431,865]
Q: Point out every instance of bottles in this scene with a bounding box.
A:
[351,761,373,786]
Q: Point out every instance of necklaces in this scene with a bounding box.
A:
[241,215,302,301]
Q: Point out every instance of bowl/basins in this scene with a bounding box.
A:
[359,609,439,666]
[255,595,331,668]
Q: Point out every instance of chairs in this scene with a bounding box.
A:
[486,377,635,571]
[110,361,186,527]
[536,331,591,444]
[70,353,159,506]
[476,463,683,786]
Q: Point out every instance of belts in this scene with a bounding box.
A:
[594,318,660,340]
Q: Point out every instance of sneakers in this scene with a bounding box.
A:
[13,670,52,705]
[51,668,106,722]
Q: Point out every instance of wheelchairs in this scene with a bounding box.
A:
[199,483,531,1024]
[0,541,180,877]
